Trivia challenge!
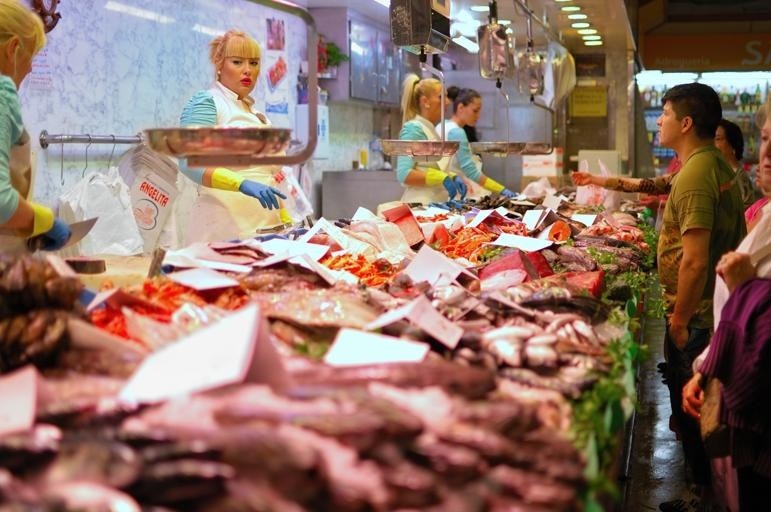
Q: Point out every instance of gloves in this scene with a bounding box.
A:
[450,168,471,202]
[209,166,287,211]
[423,166,458,202]
[9,199,73,255]
[482,177,520,198]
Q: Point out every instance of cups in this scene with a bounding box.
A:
[319,90,329,104]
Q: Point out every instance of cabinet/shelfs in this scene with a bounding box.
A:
[308,7,423,108]
[321,170,404,221]
[296,100,330,158]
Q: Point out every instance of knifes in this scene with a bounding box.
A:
[65,217,99,250]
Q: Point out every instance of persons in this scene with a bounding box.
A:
[1,0,73,259]
[394,74,467,205]
[434,85,517,200]
[651,83,749,510]
[572,116,756,211]
[176,30,314,243]
[677,93,771,511]
[745,163,771,235]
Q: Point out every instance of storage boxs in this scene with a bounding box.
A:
[521,147,563,192]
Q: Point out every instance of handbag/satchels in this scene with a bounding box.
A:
[696,376,729,445]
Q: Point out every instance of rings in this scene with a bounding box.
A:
[721,258,727,264]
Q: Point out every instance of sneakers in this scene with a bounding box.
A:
[657,362,667,373]
[655,484,713,512]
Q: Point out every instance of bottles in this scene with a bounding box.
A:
[650,155,661,167]
[718,83,764,106]
[348,129,384,170]
[639,78,667,109]
[742,136,756,157]
[723,112,754,133]
[645,112,663,148]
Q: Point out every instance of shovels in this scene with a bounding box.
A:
[28,216,98,252]
